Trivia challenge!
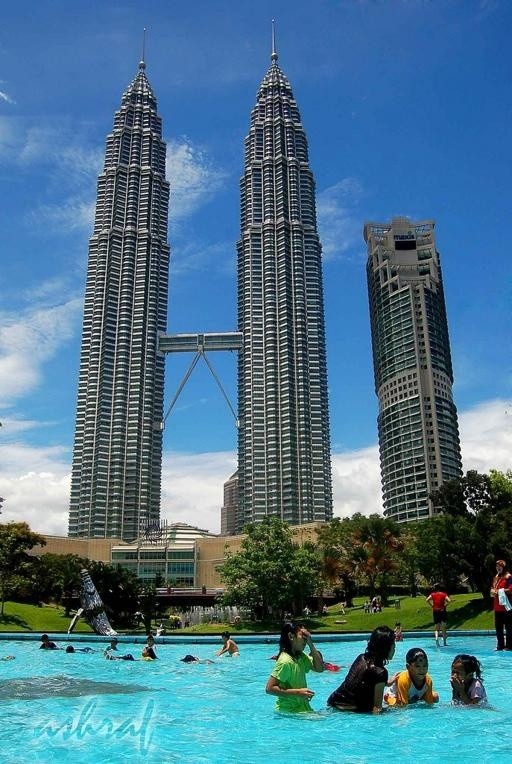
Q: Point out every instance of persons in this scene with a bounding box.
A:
[266,623,491,717]
[303,598,381,615]
[491,560,511,653]
[426,582,453,646]
[284,612,297,623]
[40,628,243,664]
